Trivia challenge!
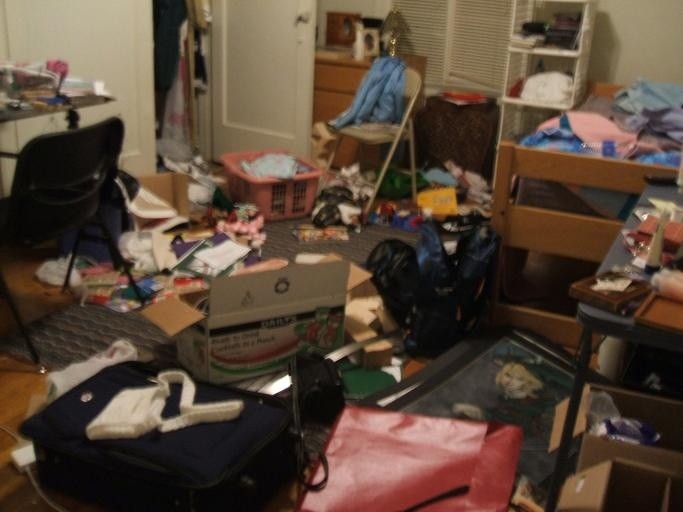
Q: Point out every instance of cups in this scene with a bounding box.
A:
[594,334,627,382]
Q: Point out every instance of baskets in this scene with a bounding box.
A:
[219,148,322,222]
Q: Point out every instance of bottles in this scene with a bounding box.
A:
[353,21,365,62]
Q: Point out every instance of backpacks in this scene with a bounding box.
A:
[401,209,503,358]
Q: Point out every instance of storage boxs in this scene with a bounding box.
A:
[136,254,375,386]
[553,456,674,512]
[548,377,683,472]
[134,171,204,218]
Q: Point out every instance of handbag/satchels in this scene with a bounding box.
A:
[288,350,347,426]
[365,237,420,321]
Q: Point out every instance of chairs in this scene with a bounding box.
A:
[321,66,423,223]
[0,116,144,363]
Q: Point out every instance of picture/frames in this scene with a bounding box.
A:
[565,269,651,312]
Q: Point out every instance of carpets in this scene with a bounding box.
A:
[0,213,458,373]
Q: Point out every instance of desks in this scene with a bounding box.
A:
[538,184,683,510]
[1,88,118,199]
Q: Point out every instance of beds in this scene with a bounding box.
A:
[486,80,683,355]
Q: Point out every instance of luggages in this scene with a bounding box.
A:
[19,359,304,511]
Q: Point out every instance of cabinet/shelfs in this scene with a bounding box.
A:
[311,49,375,126]
[491,0,594,194]
